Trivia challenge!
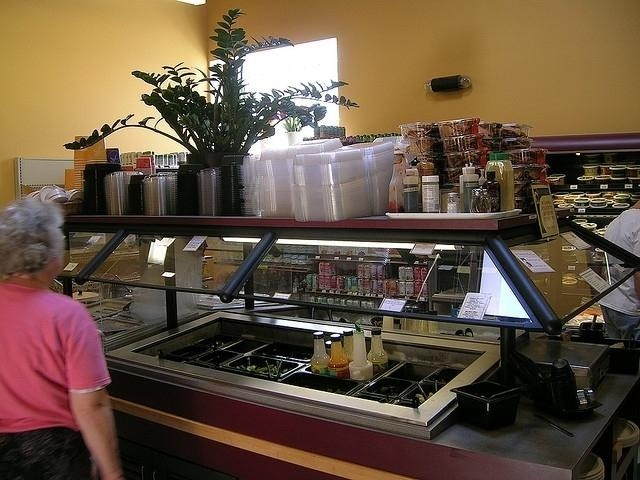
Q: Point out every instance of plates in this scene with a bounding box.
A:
[575,455,605,480]
[614,419,640,449]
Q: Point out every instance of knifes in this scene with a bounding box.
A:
[533,414,574,437]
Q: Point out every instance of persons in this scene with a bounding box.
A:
[596,197,640,341]
[0,201,124,480]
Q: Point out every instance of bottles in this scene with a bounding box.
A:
[367,328,389,372]
[390,145,515,213]
[350,331,373,381]
[327,334,350,379]
[311,331,330,375]
[341,331,354,361]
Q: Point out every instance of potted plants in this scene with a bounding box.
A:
[62,6,361,215]
[279,116,303,143]
